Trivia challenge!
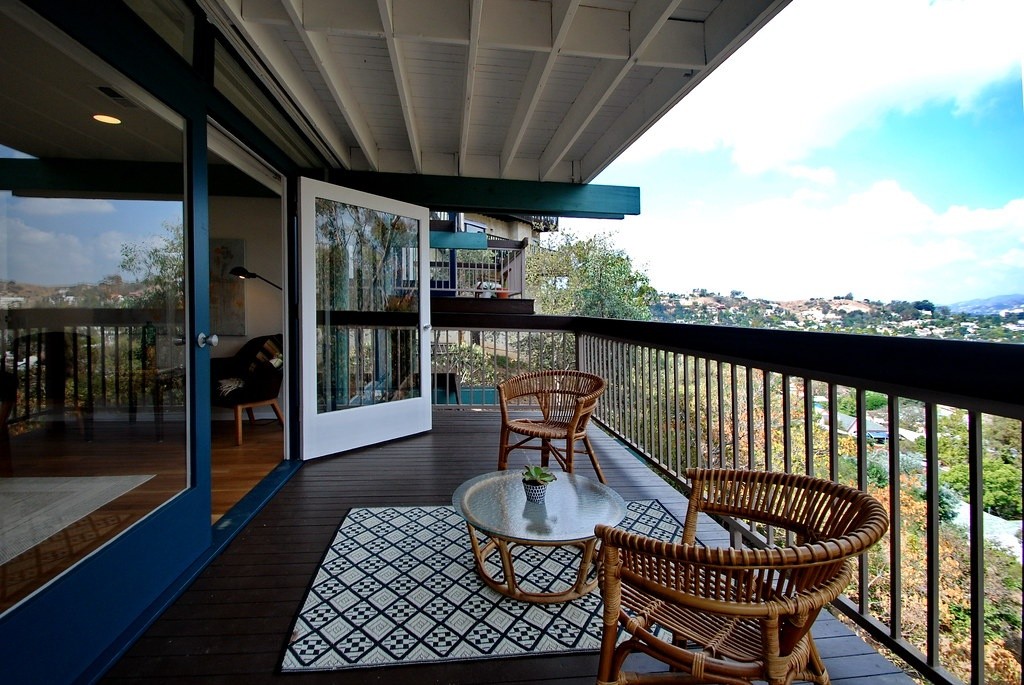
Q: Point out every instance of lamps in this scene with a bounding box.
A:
[229,266,283,292]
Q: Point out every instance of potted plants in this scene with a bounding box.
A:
[521,465,557,503]
[497,281,508,298]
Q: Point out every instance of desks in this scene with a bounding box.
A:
[452,469,630,604]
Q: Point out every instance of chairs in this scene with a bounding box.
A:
[591,468,889,685]
[473,267,515,297]
[497,370,606,490]
[208,332,284,444]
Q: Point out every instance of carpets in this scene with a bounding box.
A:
[0,474,157,568]
[282,506,765,676]
